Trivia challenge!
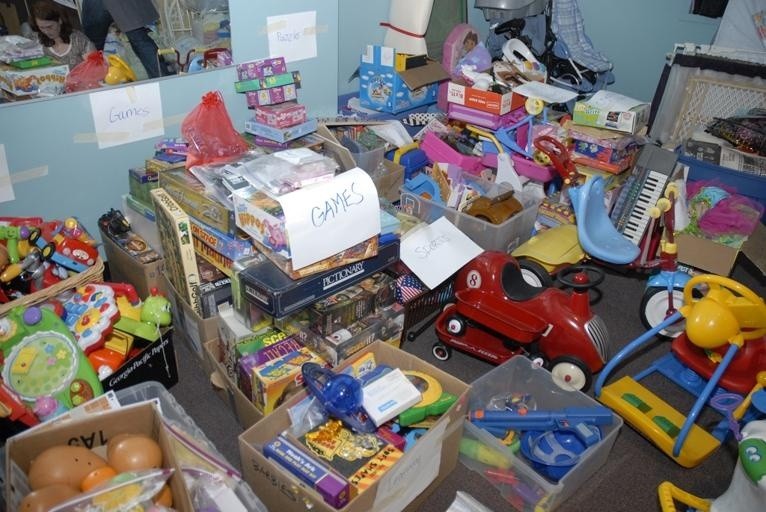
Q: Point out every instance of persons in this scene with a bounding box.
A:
[28,1,160,79]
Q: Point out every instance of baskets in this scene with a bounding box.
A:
[0,255,105,319]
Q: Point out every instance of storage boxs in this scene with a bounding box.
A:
[11,380,268,509]
[98,320,180,390]
[4,398,192,510]
[99,55,625,372]
[239,340,474,512]
[458,354,624,511]
